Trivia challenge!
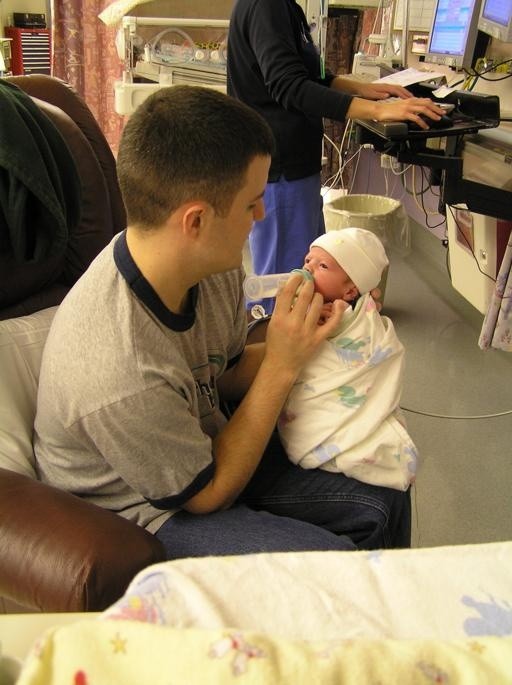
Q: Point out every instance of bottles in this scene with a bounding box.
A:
[243,269,314,301]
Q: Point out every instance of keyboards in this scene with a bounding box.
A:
[378,97,455,115]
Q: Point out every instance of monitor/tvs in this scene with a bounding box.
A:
[477,0,512,42]
[424,0,482,69]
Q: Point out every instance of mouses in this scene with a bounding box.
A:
[408,116,454,130]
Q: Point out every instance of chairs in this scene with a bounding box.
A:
[0,72,168,613]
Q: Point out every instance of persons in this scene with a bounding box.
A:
[226,0,447,316]
[301,227,389,305]
[31,86,412,560]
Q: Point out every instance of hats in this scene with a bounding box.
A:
[310,229,389,296]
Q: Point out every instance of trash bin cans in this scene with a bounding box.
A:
[323,194,402,312]
